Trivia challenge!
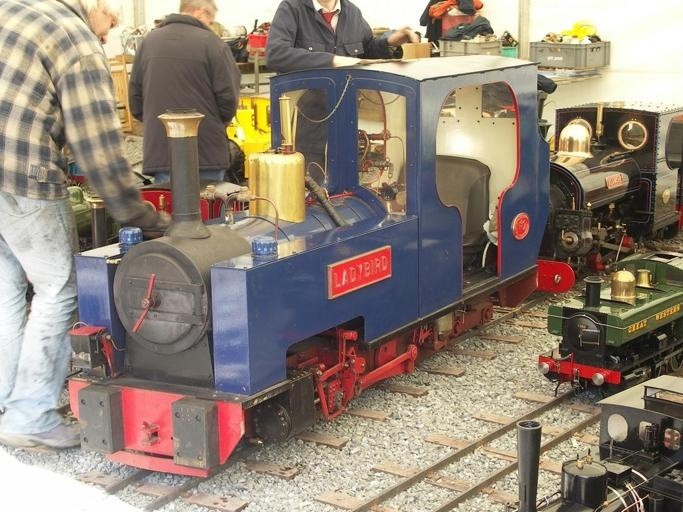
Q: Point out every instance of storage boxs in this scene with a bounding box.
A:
[439,40,502,57]
[529,41,610,69]
[357,42,431,123]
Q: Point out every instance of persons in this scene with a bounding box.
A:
[429,1,485,40]
[0,0,176,456]
[128,0,241,182]
[264,2,419,183]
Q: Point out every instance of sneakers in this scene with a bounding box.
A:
[0,420,85,451]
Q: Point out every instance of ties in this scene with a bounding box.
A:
[322,11,335,33]
